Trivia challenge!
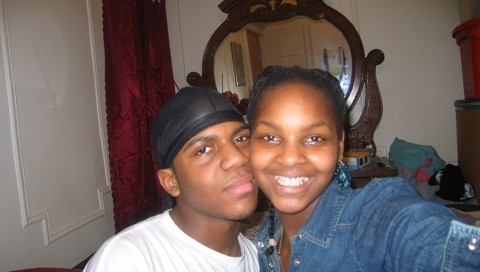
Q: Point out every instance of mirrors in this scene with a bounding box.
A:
[214,16,353,108]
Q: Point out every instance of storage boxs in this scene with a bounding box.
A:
[451,16,480,100]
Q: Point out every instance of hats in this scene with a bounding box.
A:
[150,86,246,170]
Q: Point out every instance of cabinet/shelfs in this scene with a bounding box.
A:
[454,101,480,208]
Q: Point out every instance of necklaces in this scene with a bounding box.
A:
[273,207,316,271]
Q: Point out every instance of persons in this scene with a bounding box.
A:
[79,85,264,271]
[242,65,480,271]
